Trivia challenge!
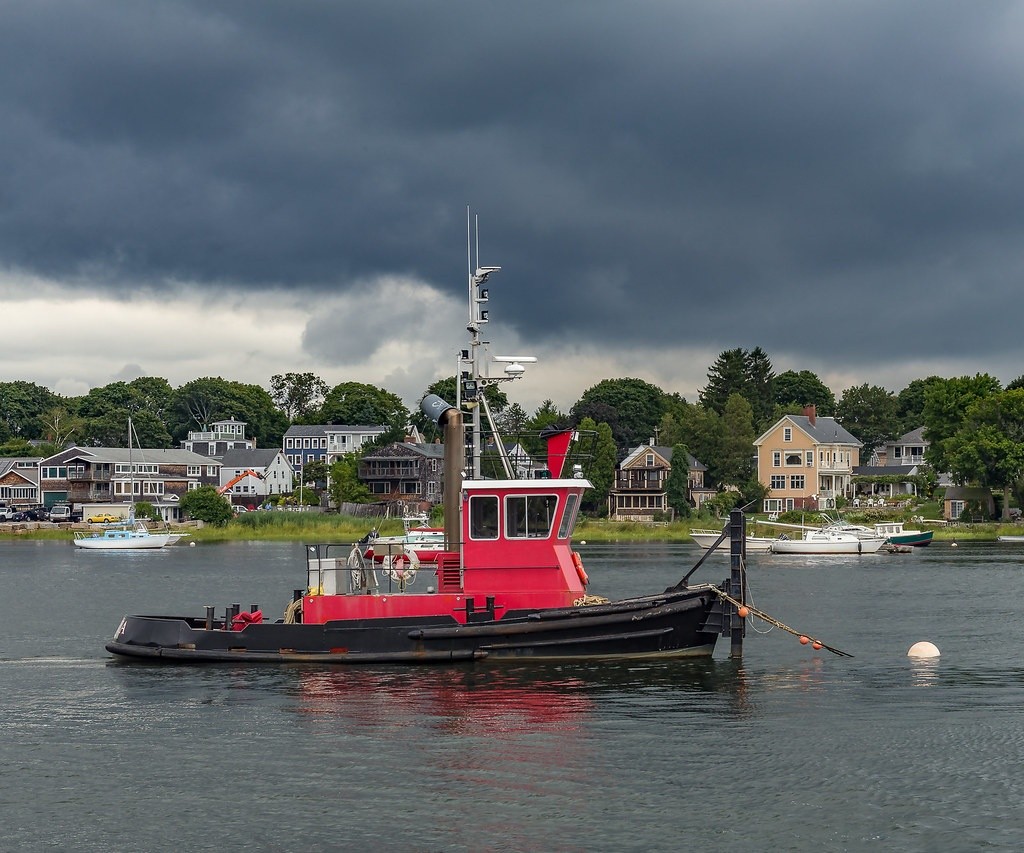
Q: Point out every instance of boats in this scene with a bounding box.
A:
[106,203,747,671]
[870,522,935,547]
[689,528,773,552]
[362,504,446,563]
[770,519,888,556]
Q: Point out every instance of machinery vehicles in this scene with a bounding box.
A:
[216,469,267,496]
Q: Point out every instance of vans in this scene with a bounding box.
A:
[0,508,13,522]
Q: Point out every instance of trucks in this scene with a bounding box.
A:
[50,505,81,523]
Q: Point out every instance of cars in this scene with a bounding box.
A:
[87,513,120,524]
[231,504,258,514]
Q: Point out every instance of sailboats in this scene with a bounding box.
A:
[73,416,190,553]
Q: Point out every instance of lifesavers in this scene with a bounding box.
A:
[382,549,419,580]
